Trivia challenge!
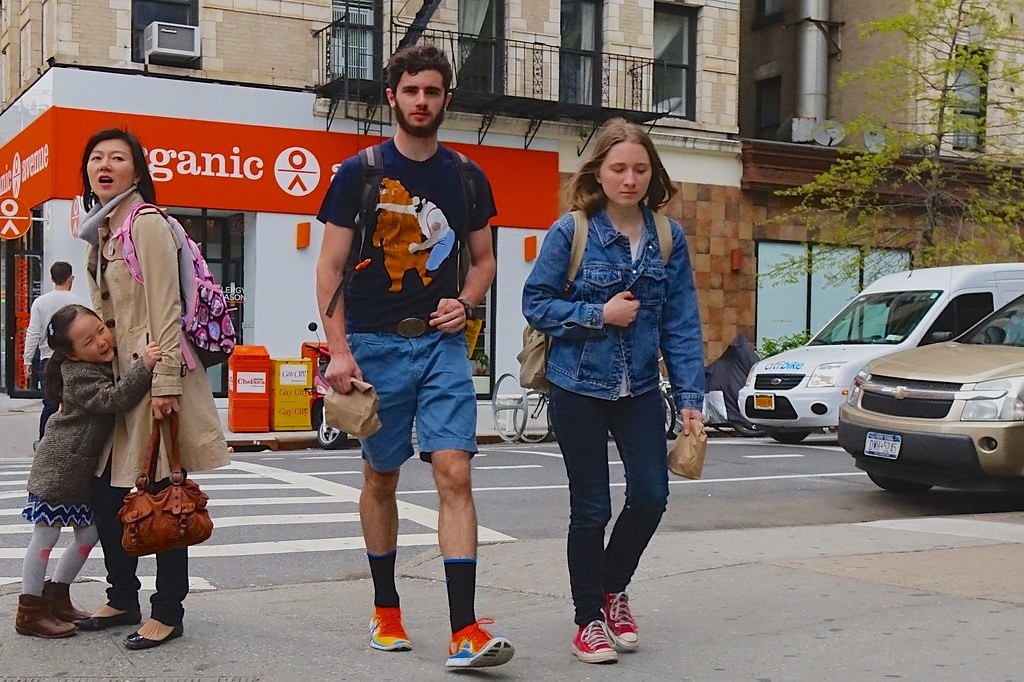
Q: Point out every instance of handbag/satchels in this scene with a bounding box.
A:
[119,410,215,555]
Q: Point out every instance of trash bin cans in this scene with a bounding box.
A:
[270,357,313,430]
[302,342,328,371]
[227,345,270,433]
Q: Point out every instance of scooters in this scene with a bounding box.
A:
[302,322,347,449]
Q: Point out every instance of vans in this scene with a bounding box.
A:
[738,263,1024,443]
[838,293,1024,491]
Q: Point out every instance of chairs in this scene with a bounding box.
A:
[947,319,969,332]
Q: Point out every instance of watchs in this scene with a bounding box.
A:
[458,299,474,320]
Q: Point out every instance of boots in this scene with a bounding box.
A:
[43,579,92,622]
[14,593,77,637]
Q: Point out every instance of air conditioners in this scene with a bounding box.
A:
[143,21,201,64]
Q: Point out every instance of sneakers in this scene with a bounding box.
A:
[602,592,639,650]
[572,620,619,663]
[446,617,515,668]
[368,607,413,650]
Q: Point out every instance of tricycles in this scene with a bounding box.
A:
[513,333,760,443]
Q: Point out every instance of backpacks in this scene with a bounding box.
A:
[515,211,672,393]
[111,204,238,370]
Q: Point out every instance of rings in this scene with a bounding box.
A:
[166,409,171,413]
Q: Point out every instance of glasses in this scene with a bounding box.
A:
[70,275,74,281]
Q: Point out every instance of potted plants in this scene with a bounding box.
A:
[475,353,488,375]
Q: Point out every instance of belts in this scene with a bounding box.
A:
[349,317,438,337]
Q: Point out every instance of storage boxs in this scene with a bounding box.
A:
[227,346,312,432]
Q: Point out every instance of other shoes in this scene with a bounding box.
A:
[33,441,39,452]
[123,624,183,649]
[73,609,142,630]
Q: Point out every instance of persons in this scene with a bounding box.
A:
[73,127,231,650]
[522,118,707,663]
[15,304,162,638]
[24,261,85,451]
[316,45,514,668]
